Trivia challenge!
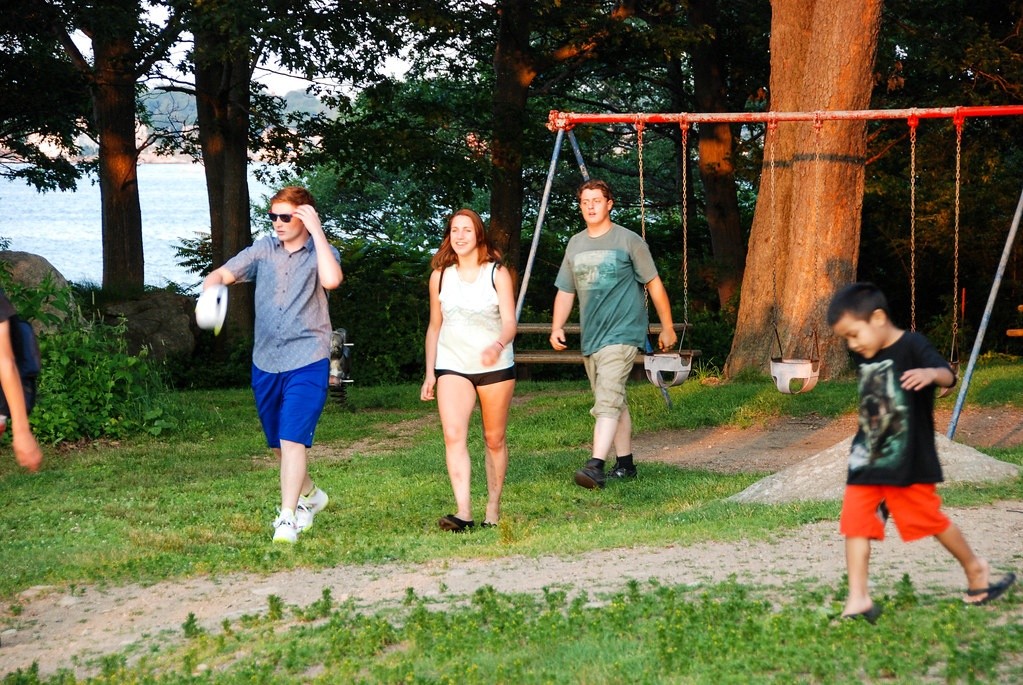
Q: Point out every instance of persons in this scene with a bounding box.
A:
[421,208,517,530]
[203,187,343,544]
[828,282,992,624]
[0,288,44,470]
[549,179,677,490]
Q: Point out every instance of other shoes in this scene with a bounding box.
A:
[481,522,496,528]
[439,516,473,532]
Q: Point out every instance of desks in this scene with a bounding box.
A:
[515,322,693,408]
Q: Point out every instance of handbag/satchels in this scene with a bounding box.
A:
[0,321,40,417]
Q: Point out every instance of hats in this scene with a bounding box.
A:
[195,286,228,337]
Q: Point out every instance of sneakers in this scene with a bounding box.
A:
[606,461,638,481]
[294,483,328,533]
[575,461,605,488]
[272,509,297,546]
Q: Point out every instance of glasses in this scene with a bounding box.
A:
[268,211,296,223]
[578,198,607,209]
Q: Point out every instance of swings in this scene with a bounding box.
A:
[766,109,825,395]
[633,111,691,389]
[907,105,964,399]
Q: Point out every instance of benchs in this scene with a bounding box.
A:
[513,350,701,365]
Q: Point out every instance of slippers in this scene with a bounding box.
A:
[966,573,1016,605]
[836,604,881,628]
